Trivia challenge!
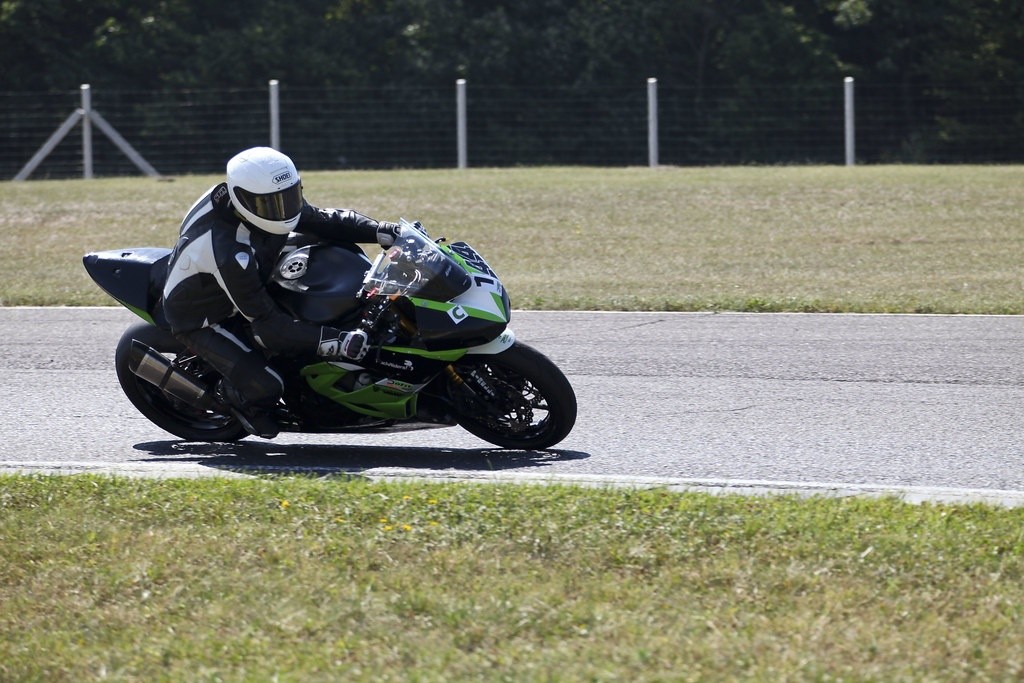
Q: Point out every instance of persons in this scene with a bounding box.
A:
[161,146,429,437]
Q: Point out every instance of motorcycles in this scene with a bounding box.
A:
[82,217,578,451]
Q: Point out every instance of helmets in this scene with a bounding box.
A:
[227,146,303,235]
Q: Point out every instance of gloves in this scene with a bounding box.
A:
[376,219,430,251]
[315,325,373,362]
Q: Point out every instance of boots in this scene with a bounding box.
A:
[215,378,282,439]
